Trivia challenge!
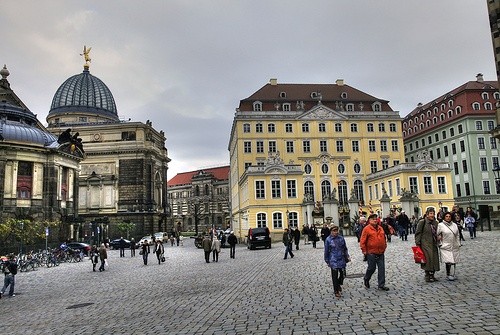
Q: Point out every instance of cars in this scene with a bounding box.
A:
[68,243,88,250]
[111,239,131,243]
[138,236,154,246]
[245,227,272,250]
[155,232,168,243]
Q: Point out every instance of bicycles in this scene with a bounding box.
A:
[0,246,81,274]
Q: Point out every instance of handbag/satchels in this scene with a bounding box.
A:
[411,246,426,263]
[139,251,143,255]
[161,257,165,262]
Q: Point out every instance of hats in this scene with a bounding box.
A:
[369,214,377,218]
[330,226,338,231]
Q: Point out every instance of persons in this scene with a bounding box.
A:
[176,233,184,247]
[152,240,166,265]
[118,236,125,258]
[141,240,150,265]
[324,225,351,297]
[360,214,390,291]
[170,235,175,247]
[282,221,336,262]
[0,253,18,298]
[227,230,238,260]
[90,242,108,272]
[129,237,136,257]
[353,206,479,282]
[204,227,227,264]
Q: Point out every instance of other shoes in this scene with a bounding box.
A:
[9,294,16,297]
[0,292,3,298]
[424,275,438,282]
[446,275,457,281]
[364,276,370,288]
[335,290,340,297]
[377,285,389,291]
